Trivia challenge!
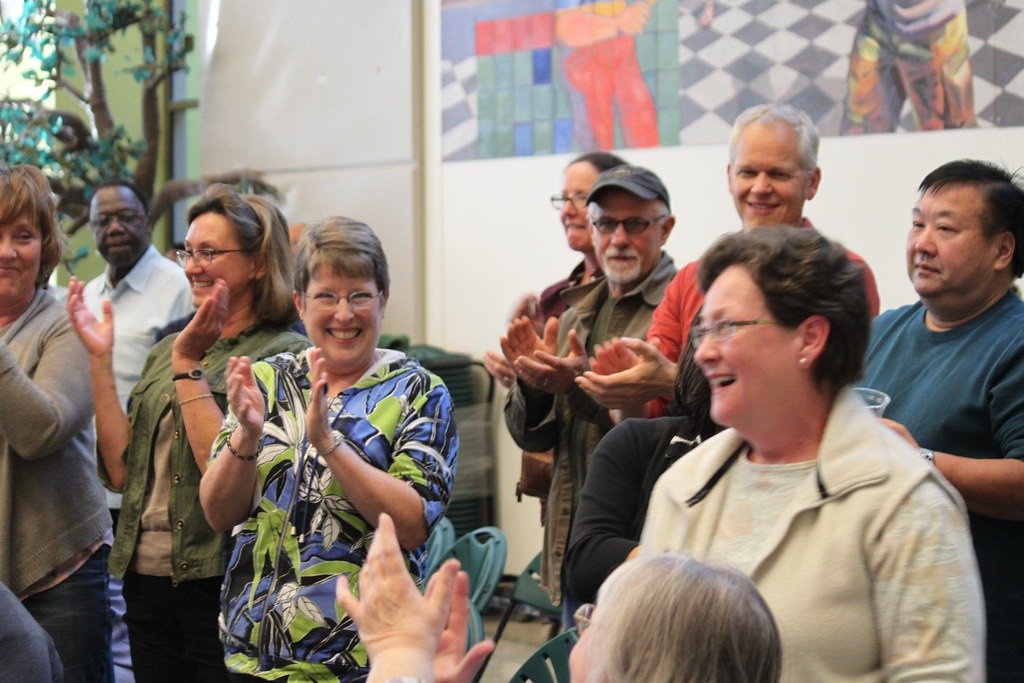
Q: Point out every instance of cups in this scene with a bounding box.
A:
[852,388,891,419]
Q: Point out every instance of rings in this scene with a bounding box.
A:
[504,375,509,382]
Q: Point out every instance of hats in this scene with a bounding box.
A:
[585,164,669,212]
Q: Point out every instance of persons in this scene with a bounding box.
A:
[635,159,1024,683]
[575,103,880,425]
[483,151,678,629]
[0,163,460,682]
[337,512,784,683]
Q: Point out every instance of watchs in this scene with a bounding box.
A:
[173,367,204,381]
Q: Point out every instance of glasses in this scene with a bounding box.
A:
[297,291,385,312]
[176,247,257,269]
[591,213,671,235]
[572,602,606,636]
[552,194,590,210]
[689,320,804,349]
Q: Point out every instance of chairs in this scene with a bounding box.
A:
[419,526,581,683]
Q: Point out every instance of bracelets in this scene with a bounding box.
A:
[318,429,345,456]
[179,392,215,405]
[919,448,935,463]
[227,432,262,461]
